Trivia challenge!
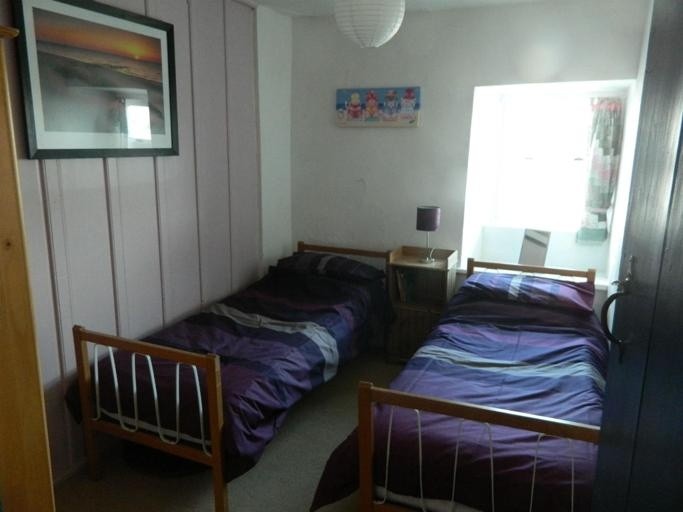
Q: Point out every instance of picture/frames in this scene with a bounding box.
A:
[9,0,181,160]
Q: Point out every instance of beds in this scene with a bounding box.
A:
[72,240,392,511]
[358,256,604,512]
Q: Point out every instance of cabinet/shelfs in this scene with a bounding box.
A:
[386,244,457,365]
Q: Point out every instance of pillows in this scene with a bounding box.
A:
[446,272,596,314]
[272,248,386,287]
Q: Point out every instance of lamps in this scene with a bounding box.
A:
[333,0,405,48]
[415,205,441,264]
